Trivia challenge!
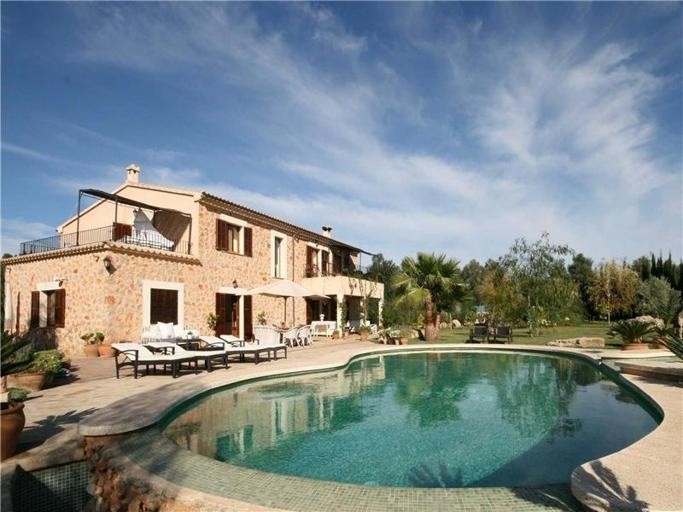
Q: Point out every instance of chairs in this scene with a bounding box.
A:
[110,324,317,381]
[467,326,514,344]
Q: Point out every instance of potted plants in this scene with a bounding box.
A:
[80,333,105,358]
[357,324,372,342]
[378,329,411,346]
[0,331,52,462]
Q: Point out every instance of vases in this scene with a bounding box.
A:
[98,344,114,358]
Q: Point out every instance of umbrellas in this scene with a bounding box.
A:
[238,277,332,323]
